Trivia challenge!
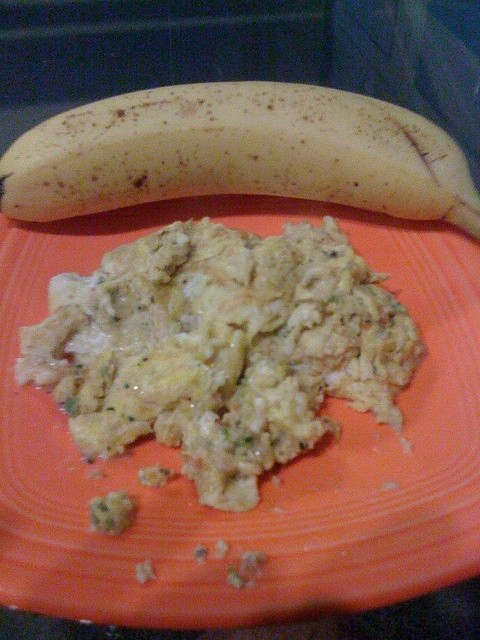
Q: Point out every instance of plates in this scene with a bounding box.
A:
[1,193,480,628]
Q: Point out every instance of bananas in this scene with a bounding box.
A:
[1,79,479,241]
